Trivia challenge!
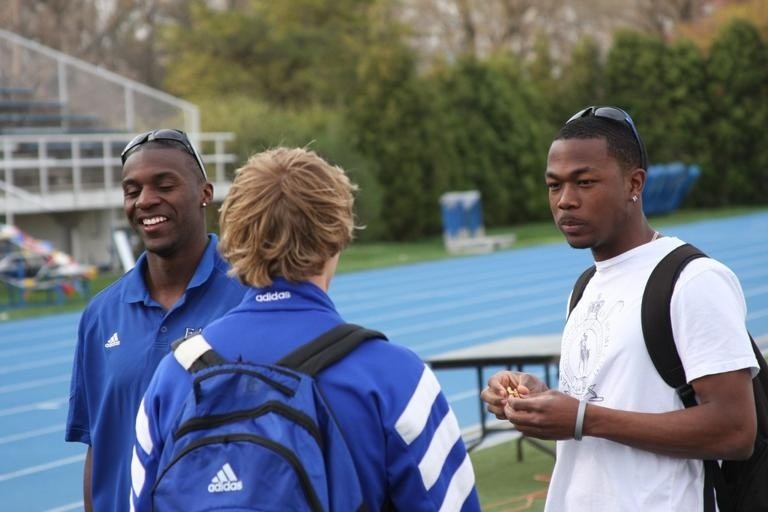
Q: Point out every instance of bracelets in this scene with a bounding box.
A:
[573,399,587,441]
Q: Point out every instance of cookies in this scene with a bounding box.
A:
[506,386,520,399]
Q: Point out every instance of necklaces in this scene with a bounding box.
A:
[650,231,659,243]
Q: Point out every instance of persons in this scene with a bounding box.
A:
[126,147,481,512]
[479,106,761,512]
[65,128,251,511]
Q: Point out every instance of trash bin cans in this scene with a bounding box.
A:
[443,189,483,226]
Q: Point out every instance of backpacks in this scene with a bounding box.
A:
[151,325,389,512]
[568,244,768,512]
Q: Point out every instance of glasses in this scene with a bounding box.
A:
[565,105,645,169]
[122,128,209,182]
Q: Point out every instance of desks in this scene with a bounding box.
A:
[421,338,566,463]
